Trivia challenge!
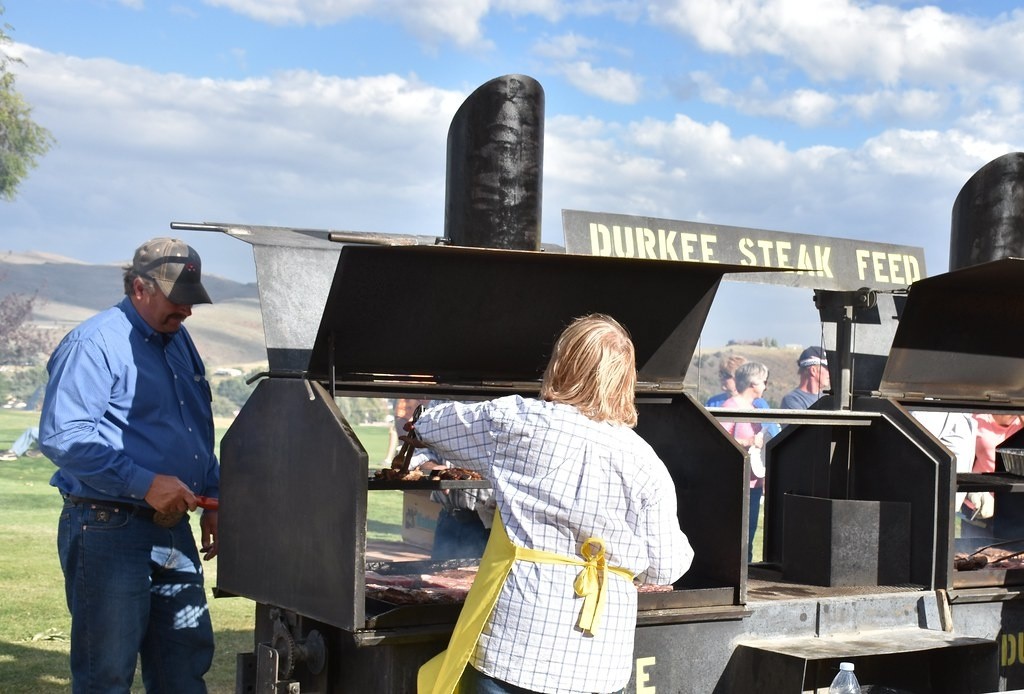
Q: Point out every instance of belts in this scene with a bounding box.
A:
[67,492,154,516]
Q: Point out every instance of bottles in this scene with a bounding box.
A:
[828,662,862,694]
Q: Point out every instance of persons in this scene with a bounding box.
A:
[377,398,428,466]
[38,237,221,694]
[705,355,781,438]
[908,411,994,519]
[719,362,770,562]
[780,346,830,429]
[398,400,496,560]
[417,314,695,694]
[0,427,45,461]
[959,414,1024,538]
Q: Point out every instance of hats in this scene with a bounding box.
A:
[798,346,829,367]
[133,238,212,305]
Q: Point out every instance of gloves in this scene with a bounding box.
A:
[968,489,994,519]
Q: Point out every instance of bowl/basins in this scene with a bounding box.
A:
[995,448,1024,477]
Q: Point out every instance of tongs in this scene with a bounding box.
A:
[391,403,424,479]
[968,538,1024,564]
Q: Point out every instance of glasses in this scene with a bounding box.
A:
[719,369,735,381]
